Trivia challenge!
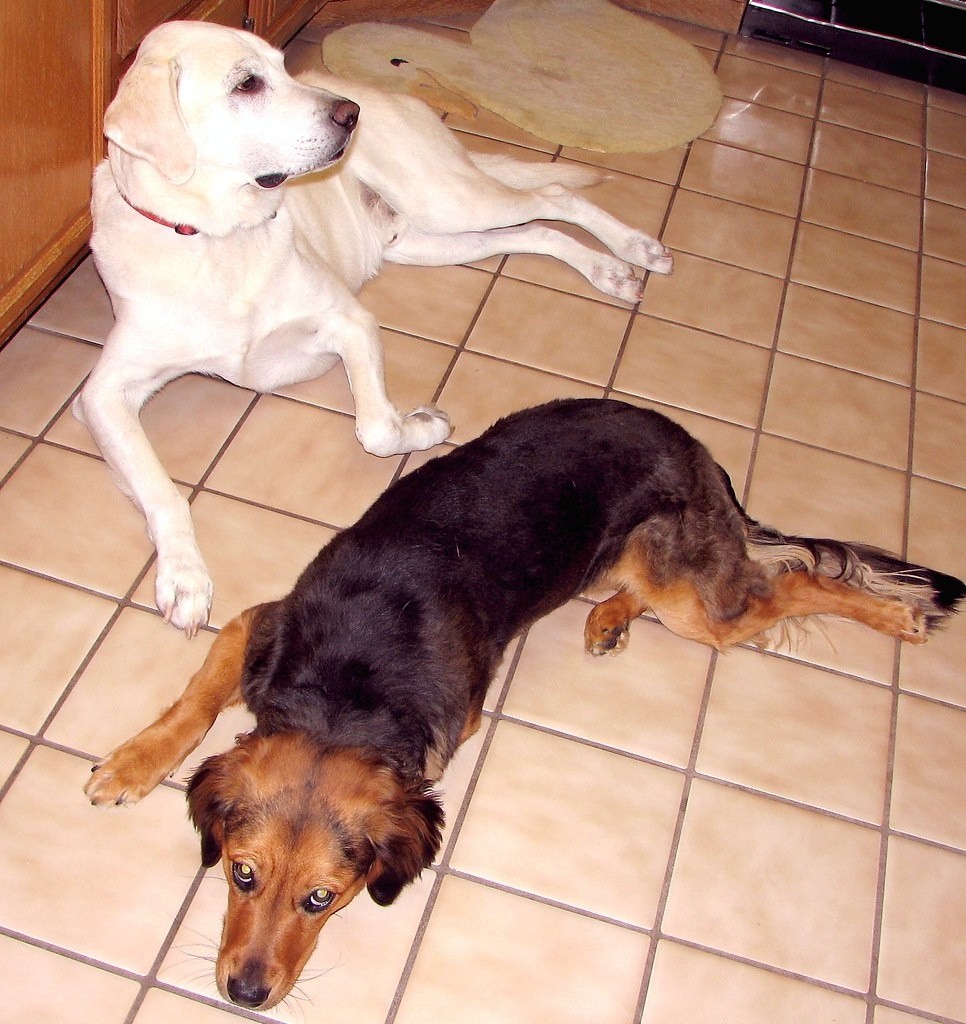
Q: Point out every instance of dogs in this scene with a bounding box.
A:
[71,20,674,642]
[84,397,966,1014]
